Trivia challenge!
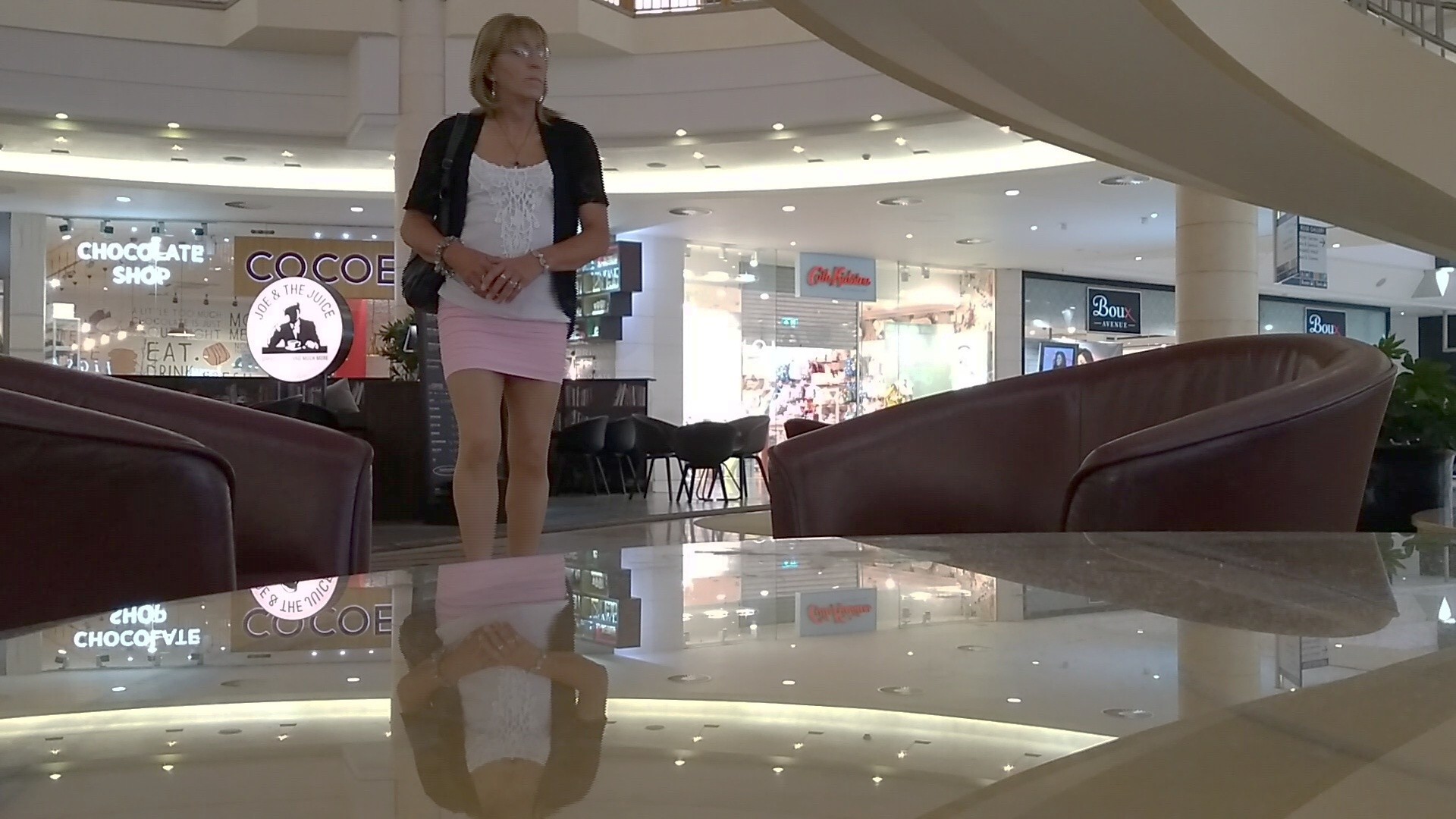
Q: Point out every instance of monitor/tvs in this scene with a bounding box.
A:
[1036,342,1079,372]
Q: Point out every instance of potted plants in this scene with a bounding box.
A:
[1359,333,1456,533]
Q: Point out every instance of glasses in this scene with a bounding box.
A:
[500,43,552,61]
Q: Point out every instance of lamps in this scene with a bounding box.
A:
[56,216,76,242]
[99,218,116,239]
[365,298,394,359]
[148,221,167,241]
[190,222,210,243]
[166,241,196,340]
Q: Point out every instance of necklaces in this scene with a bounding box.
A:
[495,113,536,169]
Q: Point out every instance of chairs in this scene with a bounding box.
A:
[764,331,1401,539]
[0,353,377,633]
[546,411,771,505]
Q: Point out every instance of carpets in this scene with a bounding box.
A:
[693,511,774,537]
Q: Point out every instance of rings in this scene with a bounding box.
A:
[501,273,507,280]
[498,631,522,650]
[470,284,475,292]
[516,283,522,292]
[510,281,516,285]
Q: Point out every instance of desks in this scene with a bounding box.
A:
[0,524,1456,819]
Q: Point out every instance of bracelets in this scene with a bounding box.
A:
[427,647,458,690]
[528,650,547,676]
[530,250,549,273]
[434,236,464,279]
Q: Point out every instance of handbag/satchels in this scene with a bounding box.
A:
[401,113,470,314]
[755,350,856,441]
[397,610,458,714]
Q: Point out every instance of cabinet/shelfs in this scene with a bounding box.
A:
[45,315,83,372]
[551,377,658,433]
[560,546,644,652]
[565,239,644,344]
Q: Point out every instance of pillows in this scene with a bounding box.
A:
[310,378,360,413]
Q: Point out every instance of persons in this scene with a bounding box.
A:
[400,13,608,561]
[1077,348,1094,365]
[1052,351,1067,369]
[394,562,609,819]
